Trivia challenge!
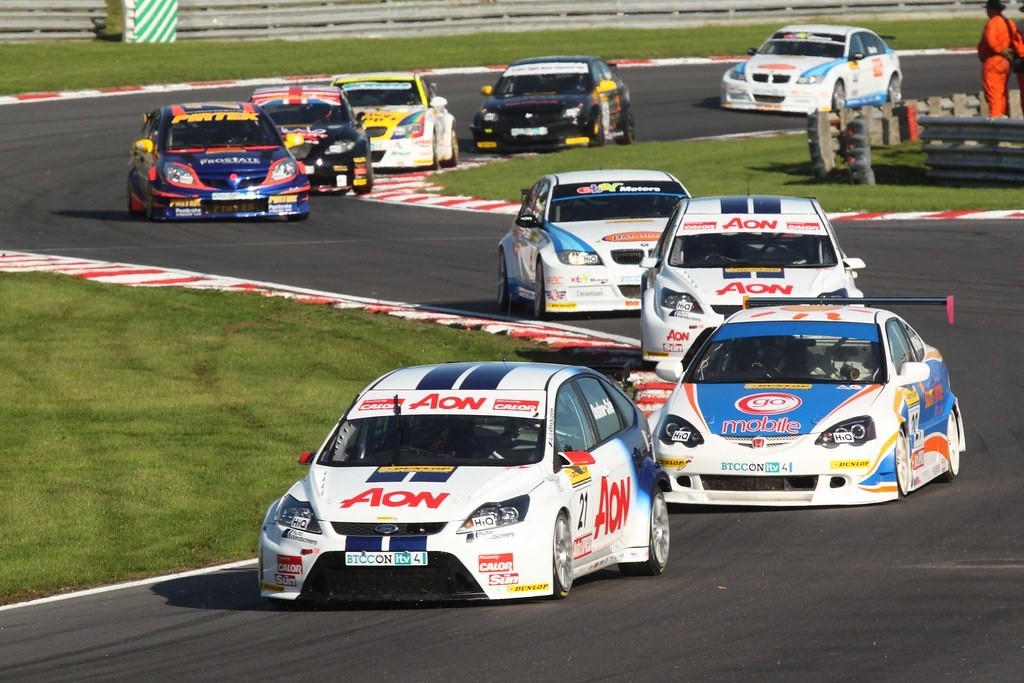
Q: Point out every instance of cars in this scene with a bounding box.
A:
[124,100,313,221]
[649,301,967,507]
[492,167,692,319]
[329,70,461,173]
[244,79,370,196]
[259,360,674,607]
[640,193,863,368]
[471,57,638,155]
[717,21,905,117]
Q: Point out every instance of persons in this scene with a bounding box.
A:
[224,118,268,146]
[748,333,809,379]
[977,0,1024,121]
[405,415,469,463]
[400,88,422,104]
[300,103,342,127]
